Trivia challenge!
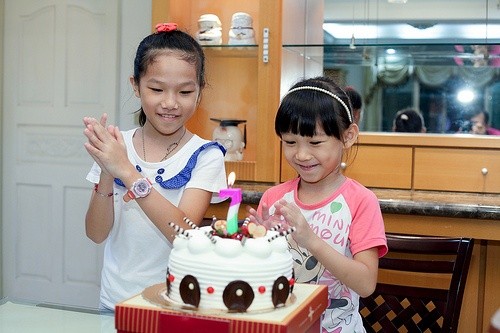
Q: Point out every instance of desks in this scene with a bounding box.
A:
[0,299,114,333]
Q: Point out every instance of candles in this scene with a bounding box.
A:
[219,172,242,235]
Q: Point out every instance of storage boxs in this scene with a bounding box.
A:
[115,280,328,333]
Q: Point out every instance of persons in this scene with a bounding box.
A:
[250,77,389,333]
[457,106,500,136]
[83,30,227,314]
[392,108,427,132]
[344,86,362,125]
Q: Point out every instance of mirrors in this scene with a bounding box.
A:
[324,0,500,136]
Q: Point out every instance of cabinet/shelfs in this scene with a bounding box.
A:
[150,0,500,194]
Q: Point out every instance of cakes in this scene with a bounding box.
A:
[163,214,296,313]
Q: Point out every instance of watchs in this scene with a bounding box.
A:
[123,177,153,202]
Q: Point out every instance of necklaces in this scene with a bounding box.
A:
[142,127,187,163]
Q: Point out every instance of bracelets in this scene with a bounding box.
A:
[95,183,113,197]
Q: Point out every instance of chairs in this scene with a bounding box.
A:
[360,234,474,333]
